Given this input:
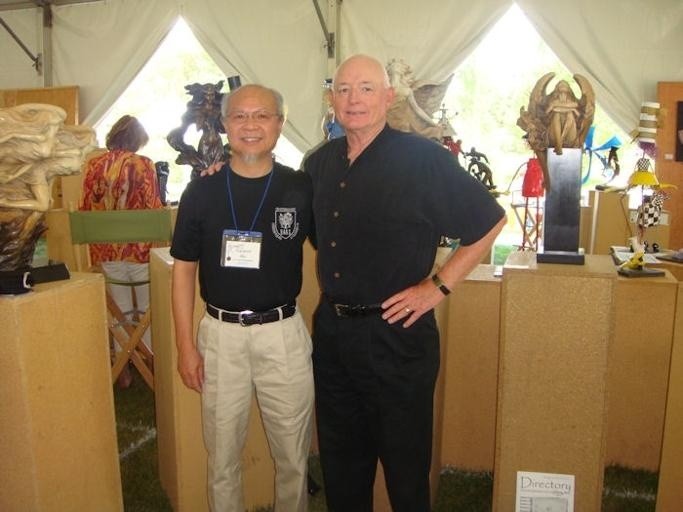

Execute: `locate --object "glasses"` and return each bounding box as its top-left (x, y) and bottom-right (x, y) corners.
top-left (227, 108), bottom-right (280, 122)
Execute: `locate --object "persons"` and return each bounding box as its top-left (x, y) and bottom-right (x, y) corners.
top-left (170, 83), bottom-right (317, 512)
top-left (515, 71), bottom-right (594, 193)
top-left (77, 115), bottom-right (164, 391)
top-left (170, 77), bottom-right (230, 182)
top-left (201, 54), bottom-right (507, 512)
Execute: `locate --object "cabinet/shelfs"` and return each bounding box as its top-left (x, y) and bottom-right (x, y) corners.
top-left (0, 272), bottom-right (127, 512)
top-left (148, 247), bottom-right (683, 511)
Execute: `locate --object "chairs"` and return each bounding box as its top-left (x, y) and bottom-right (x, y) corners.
top-left (70, 208), bottom-right (167, 394)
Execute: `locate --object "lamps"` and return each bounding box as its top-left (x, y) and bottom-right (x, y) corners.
top-left (618, 157), bottom-right (660, 245)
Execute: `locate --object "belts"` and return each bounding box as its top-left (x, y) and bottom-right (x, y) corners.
top-left (329, 301), bottom-right (384, 319)
top-left (205, 303), bottom-right (293, 327)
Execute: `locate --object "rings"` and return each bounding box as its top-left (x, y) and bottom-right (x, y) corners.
top-left (404, 307), bottom-right (411, 314)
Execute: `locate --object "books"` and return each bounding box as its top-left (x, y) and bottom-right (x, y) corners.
top-left (609, 245), bottom-right (666, 268)
top-left (656, 254), bottom-right (683, 263)
top-left (617, 267), bottom-right (666, 278)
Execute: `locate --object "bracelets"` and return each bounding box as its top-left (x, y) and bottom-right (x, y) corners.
top-left (432, 274), bottom-right (452, 295)
